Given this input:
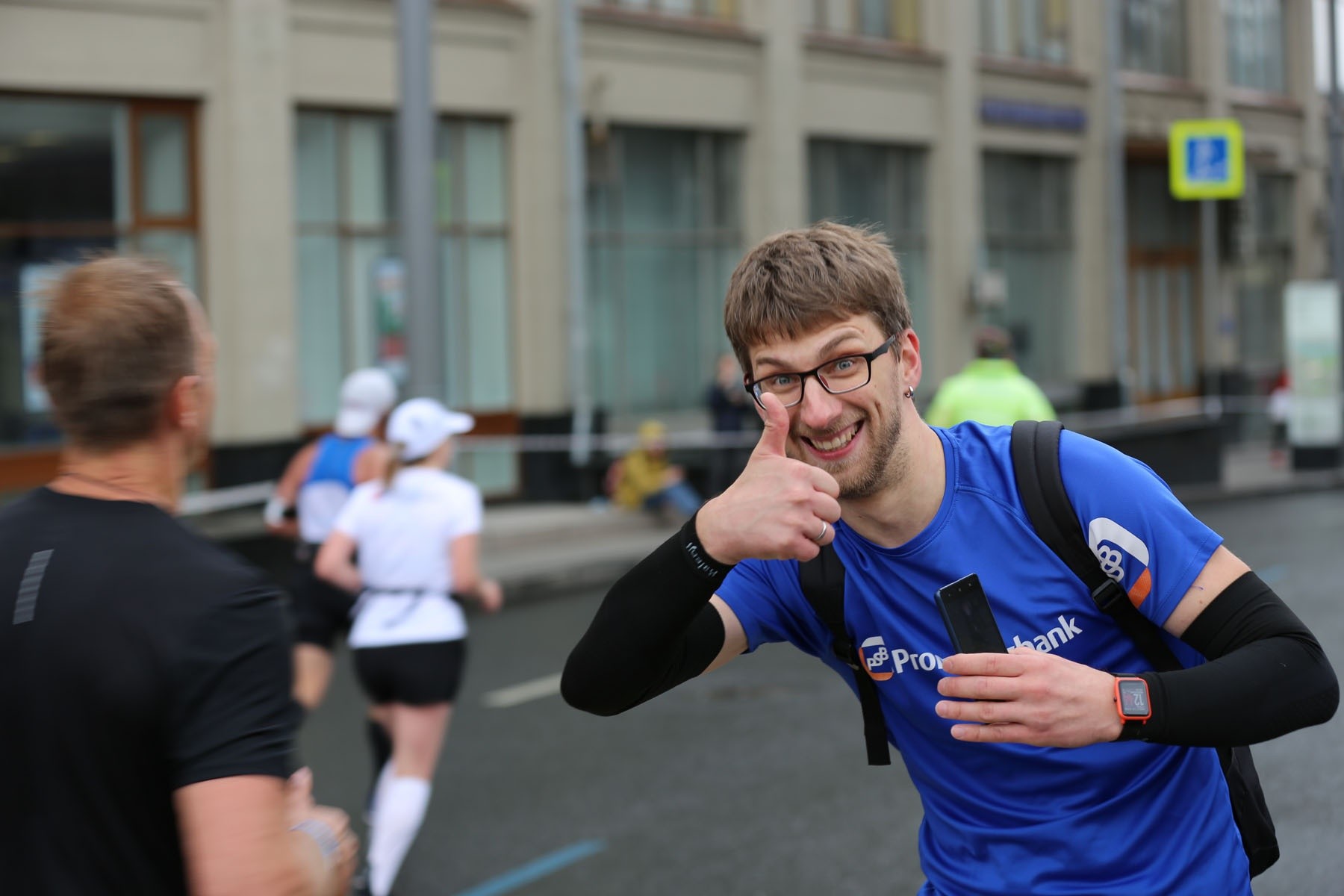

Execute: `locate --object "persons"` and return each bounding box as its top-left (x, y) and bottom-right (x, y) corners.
top-left (0, 257), bottom-right (363, 896)
top-left (707, 358), bottom-right (752, 482)
top-left (314, 397), bottom-right (502, 896)
top-left (922, 329), bottom-right (1061, 428)
top-left (559, 220), bottom-right (1340, 896)
top-left (262, 366), bottom-right (395, 822)
top-left (614, 420), bottom-right (701, 518)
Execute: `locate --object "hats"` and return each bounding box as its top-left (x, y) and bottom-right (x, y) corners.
top-left (332, 367), bottom-right (397, 438)
top-left (385, 398), bottom-right (476, 465)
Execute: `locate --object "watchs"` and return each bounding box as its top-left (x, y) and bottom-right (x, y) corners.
top-left (1114, 670), bottom-right (1153, 743)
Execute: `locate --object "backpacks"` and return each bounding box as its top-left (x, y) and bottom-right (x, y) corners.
top-left (799, 419), bottom-right (1284, 881)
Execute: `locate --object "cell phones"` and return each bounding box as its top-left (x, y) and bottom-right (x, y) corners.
top-left (933, 573), bottom-right (1010, 703)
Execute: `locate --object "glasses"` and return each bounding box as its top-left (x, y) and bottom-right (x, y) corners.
top-left (743, 334), bottom-right (896, 411)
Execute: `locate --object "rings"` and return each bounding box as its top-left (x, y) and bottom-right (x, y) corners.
top-left (814, 522), bottom-right (829, 542)
